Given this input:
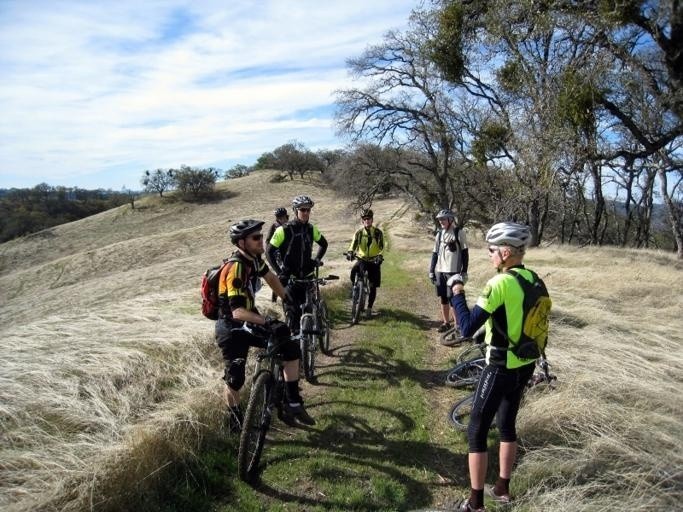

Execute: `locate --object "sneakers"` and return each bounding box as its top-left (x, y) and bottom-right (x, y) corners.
top-left (283, 403), bottom-right (315, 425)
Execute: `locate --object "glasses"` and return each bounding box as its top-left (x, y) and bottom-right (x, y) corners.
top-left (300, 208), bottom-right (310, 212)
top-left (253, 235), bottom-right (263, 240)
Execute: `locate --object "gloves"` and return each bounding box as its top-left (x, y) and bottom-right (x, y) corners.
top-left (447, 274), bottom-right (463, 288)
top-left (266, 320), bottom-right (291, 337)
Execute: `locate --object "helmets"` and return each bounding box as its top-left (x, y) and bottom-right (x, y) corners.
top-left (436, 209), bottom-right (453, 223)
top-left (361, 209), bottom-right (373, 216)
top-left (486, 222), bottom-right (530, 248)
top-left (293, 196), bottom-right (313, 212)
top-left (230, 220), bottom-right (265, 237)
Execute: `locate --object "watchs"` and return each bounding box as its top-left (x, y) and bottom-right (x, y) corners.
top-left (263, 315), bottom-right (271, 329)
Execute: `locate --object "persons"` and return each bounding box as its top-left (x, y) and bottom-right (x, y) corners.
top-left (428, 209), bottom-right (471, 332)
top-left (216, 219), bottom-right (320, 433)
top-left (263, 194), bottom-right (329, 371)
top-left (446, 220), bottom-right (540, 511)
top-left (265, 206), bottom-right (294, 304)
top-left (347, 207), bottom-right (384, 320)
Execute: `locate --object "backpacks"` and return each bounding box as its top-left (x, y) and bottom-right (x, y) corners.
top-left (494, 268), bottom-right (551, 359)
top-left (201, 257), bottom-right (242, 320)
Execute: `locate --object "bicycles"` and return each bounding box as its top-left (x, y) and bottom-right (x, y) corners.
top-left (440, 326), bottom-right (487, 366)
top-left (445, 351), bottom-right (558, 429)
top-left (343, 252), bottom-right (384, 323)
top-left (287, 275), bottom-right (339, 382)
top-left (270, 252), bottom-right (289, 318)
top-left (231, 323), bottom-right (326, 481)
